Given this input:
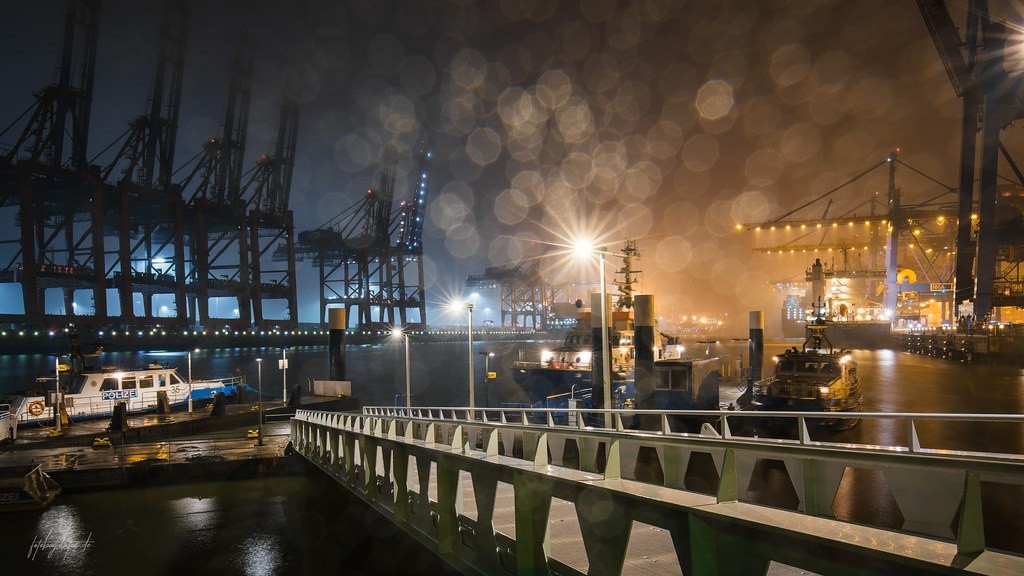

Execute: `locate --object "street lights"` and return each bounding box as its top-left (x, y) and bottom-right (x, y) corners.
top-left (444, 296), bottom-right (476, 421)
top-left (572, 242), bottom-right (613, 429)
top-left (480, 351), bottom-right (497, 407)
top-left (386, 325), bottom-right (412, 418)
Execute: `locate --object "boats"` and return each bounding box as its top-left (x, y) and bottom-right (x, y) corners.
top-left (750, 296), bottom-right (864, 435)
top-left (0, 306), bottom-right (356, 448)
top-left (511, 238), bottom-right (685, 430)
top-left (538, 292), bottom-right (767, 465)
top-left (0, 354), bottom-right (250, 428)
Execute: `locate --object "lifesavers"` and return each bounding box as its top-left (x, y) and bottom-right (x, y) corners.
top-left (28, 401), bottom-right (45, 415)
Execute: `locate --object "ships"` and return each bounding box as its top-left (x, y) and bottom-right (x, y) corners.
top-left (730, 2), bottom-right (1023, 367)
